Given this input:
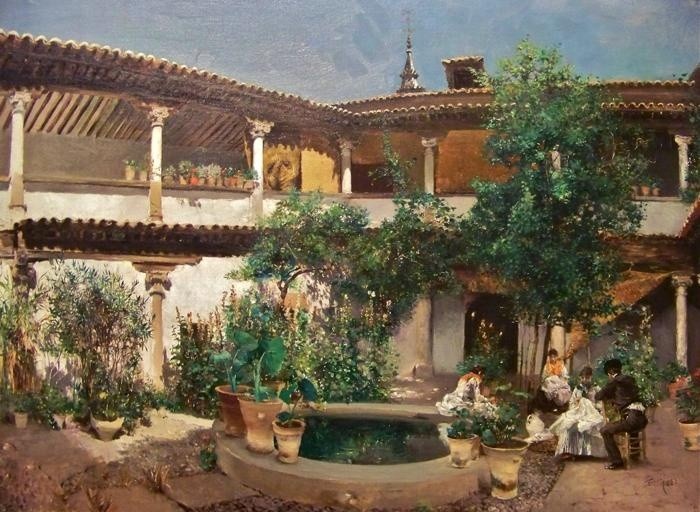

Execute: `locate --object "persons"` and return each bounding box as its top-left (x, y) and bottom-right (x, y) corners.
top-left (542, 349), bottom-right (569, 412)
top-left (594, 358), bottom-right (648, 470)
top-left (436, 366), bottom-right (500, 419)
top-left (550, 366), bottom-right (610, 460)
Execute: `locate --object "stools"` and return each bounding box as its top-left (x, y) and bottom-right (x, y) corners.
top-left (617, 429), bottom-right (646, 469)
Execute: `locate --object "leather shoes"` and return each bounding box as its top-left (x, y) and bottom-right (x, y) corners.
top-left (604, 463), bottom-right (624, 470)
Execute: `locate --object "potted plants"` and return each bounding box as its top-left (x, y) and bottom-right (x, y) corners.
top-left (455, 349), bottom-right (514, 397)
top-left (0, 259), bottom-right (154, 442)
top-left (473, 404), bottom-right (529, 500)
top-left (215, 322), bottom-right (318, 466)
top-left (676, 385), bottom-right (700, 451)
top-left (123, 159), bottom-right (256, 193)
top-left (663, 360), bottom-right (688, 400)
top-left (626, 170), bottom-right (661, 196)
top-left (444, 407), bottom-right (477, 468)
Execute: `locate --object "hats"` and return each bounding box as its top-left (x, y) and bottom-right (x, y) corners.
top-left (579, 367), bottom-right (593, 377)
top-left (604, 359), bottom-right (621, 375)
